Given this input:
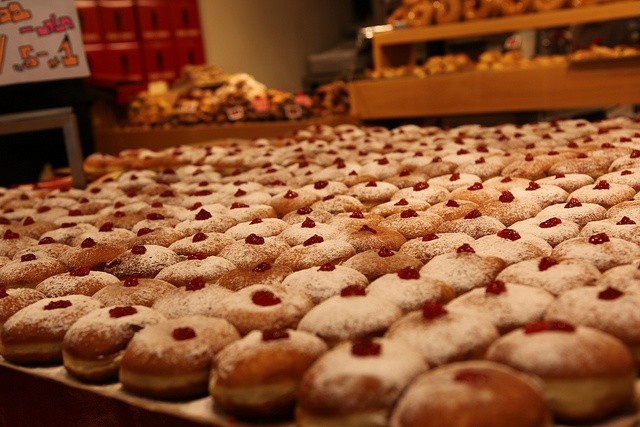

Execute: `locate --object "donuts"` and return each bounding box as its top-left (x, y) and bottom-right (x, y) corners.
top-left (387, 0), bottom-right (608, 29)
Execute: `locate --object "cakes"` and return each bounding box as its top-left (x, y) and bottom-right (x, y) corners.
top-left (0, 118), bottom-right (639, 427)
top-left (129, 59), bottom-right (347, 124)
top-left (361, 41), bottom-right (639, 81)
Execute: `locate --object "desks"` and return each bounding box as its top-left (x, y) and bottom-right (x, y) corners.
top-left (2, 104), bottom-right (84, 189)
